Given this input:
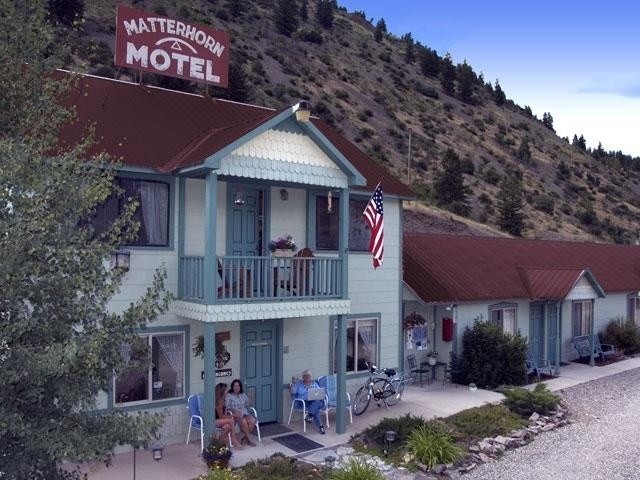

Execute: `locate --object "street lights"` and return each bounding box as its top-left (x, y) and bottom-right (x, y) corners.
top-left (133, 431), bottom-right (164, 480)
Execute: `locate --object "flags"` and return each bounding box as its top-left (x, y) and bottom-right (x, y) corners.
top-left (363, 182), bottom-right (388, 270)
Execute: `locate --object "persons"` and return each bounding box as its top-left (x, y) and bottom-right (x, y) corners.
top-left (226, 379), bottom-right (260, 446)
top-left (289, 369), bottom-right (327, 436)
top-left (212, 382), bottom-right (246, 450)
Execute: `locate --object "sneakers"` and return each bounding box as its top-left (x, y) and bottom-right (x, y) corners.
top-left (320, 425), bottom-right (326, 434)
top-left (305, 414), bottom-right (313, 423)
top-left (234, 437), bottom-right (256, 450)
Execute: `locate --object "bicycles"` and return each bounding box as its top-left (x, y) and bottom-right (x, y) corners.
top-left (351, 358), bottom-right (407, 417)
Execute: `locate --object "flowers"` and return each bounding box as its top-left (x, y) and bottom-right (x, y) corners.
top-left (269, 235), bottom-right (297, 251)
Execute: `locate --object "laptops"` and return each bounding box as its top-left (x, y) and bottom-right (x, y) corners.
top-left (304, 388), bottom-right (325, 401)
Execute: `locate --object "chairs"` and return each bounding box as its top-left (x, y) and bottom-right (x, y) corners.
top-left (523, 350), bottom-right (553, 379)
top-left (573, 334), bottom-right (616, 364)
top-left (407, 354), bottom-right (464, 390)
top-left (185, 392), bottom-right (261, 453)
top-left (216, 247), bottom-right (317, 300)
top-left (287, 374), bottom-right (353, 433)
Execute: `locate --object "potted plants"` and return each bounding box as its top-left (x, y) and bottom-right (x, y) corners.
top-left (425, 350), bottom-right (439, 366)
